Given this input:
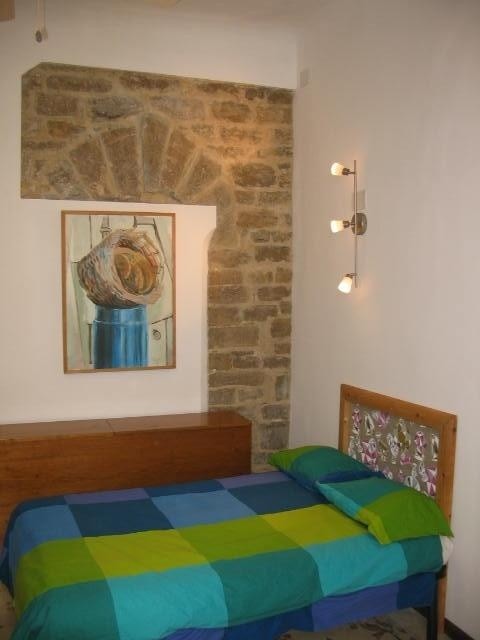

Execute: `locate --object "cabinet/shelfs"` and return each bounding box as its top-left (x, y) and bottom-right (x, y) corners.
top-left (0, 410), bottom-right (253, 538)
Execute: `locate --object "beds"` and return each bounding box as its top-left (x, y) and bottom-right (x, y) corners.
top-left (0, 384), bottom-right (458, 638)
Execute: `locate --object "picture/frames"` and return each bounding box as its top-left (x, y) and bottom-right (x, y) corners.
top-left (61, 210), bottom-right (176, 373)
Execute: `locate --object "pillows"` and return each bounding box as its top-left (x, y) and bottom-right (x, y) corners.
top-left (264, 445), bottom-right (367, 490)
top-left (316, 471), bottom-right (455, 545)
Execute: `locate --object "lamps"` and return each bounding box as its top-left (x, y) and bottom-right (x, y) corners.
top-left (330, 160), bottom-right (358, 294)
